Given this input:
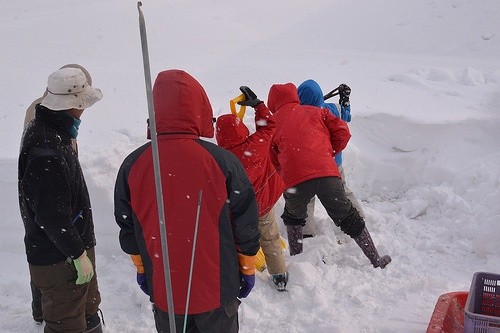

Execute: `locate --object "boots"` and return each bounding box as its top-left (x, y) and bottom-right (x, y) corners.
top-left (353, 225), bottom-right (392, 270)
top-left (286, 224), bottom-right (304, 256)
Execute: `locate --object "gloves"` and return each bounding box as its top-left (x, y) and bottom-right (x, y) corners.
top-left (236, 251), bottom-right (257, 299)
top-left (130, 254), bottom-right (150, 295)
top-left (238, 85), bottom-right (261, 108)
top-left (73, 249), bottom-right (95, 286)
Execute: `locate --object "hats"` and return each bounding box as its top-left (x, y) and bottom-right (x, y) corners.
top-left (40, 67), bottom-right (104, 111)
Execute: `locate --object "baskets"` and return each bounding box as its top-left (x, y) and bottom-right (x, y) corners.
top-left (464, 271), bottom-right (500, 333)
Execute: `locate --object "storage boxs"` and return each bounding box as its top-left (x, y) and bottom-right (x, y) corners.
top-left (463, 271), bottom-right (500, 333)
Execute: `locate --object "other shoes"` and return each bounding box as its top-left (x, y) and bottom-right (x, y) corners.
top-left (273, 271), bottom-right (288, 292)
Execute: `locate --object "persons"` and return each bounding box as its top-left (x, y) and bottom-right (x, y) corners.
top-left (18, 63), bottom-right (103, 333)
top-left (268, 82), bottom-right (392, 268)
top-left (114, 68), bottom-right (260, 333)
top-left (298, 80), bottom-right (366, 238)
top-left (214, 86), bottom-right (289, 292)
